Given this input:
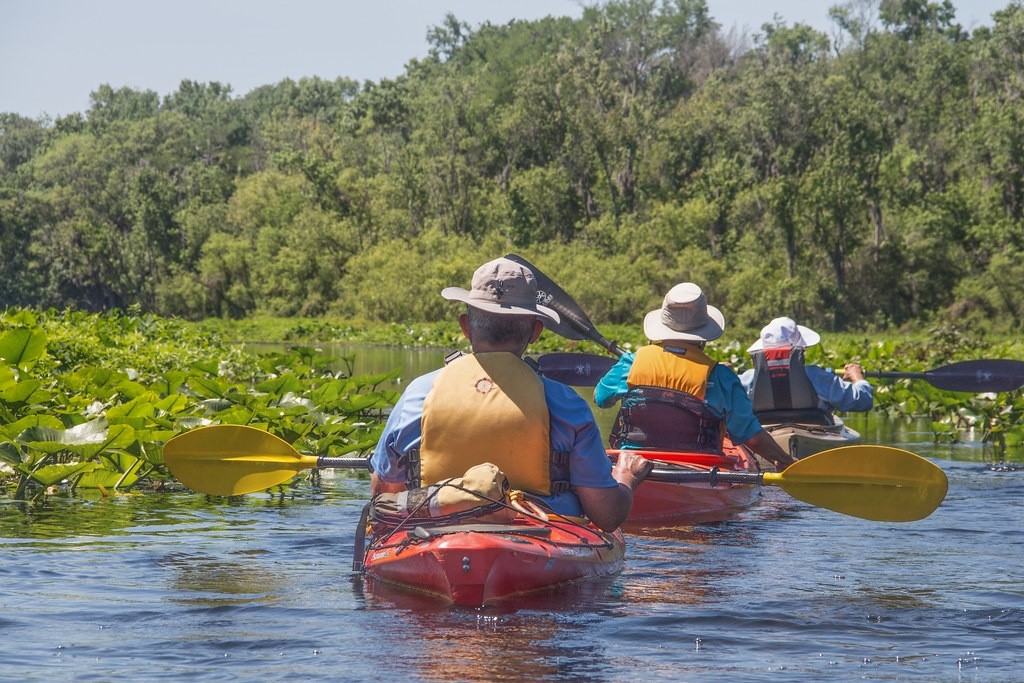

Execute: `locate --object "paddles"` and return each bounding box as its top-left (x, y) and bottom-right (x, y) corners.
top-left (502, 253), bottom-right (627, 361)
top-left (160, 422), bottom-right (952, 524)
top-left (539, 350), bottom-right (1024, 398)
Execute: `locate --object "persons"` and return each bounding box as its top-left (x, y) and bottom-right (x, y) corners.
top-left (369, 257), bottom-right (653, 533)
top-left (737, 316), bottom-right (874, 413)
top-left (593, 282), bottom-right (799, 474)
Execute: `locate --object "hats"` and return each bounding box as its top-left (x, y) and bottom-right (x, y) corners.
top-left (644, 282), bottom-right (725, 340)
top-left (746, 315), bottom-right (820, 353)
top-left (441, 258), bottom-right (561, 325)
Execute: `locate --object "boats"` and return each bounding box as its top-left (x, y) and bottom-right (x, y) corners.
top-left (602, 435), bottom-right (765, 528)
top-left (753, 412), bottom-right (863, 471)
top-left (352, 464), bottom-right (628, 611)
